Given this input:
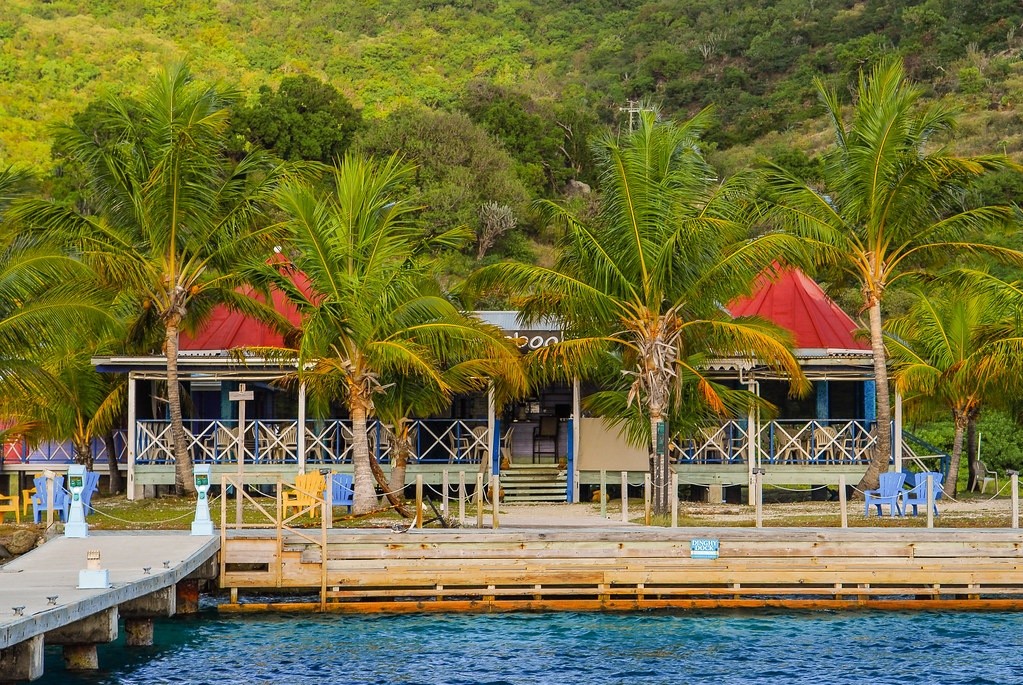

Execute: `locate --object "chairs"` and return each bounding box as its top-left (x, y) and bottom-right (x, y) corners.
top-left (446, 426), bottom-right (471, 464)
top-left (970, 460), bottom-right (998, 495)
top-left (22, 474), bottom-right (62, 516)
top-left (152, 426), bottom-right (391, 467)
top-left (282, 471), bottom-right (353, 518)
top-left (677, 423), bottom-right (878, 464)
top-left (531, 415), bottom-right (559, 463)
top-left (58, 472), bottom-right (100, 522)
top-left (500, 425), bottom-right (515, 464)
top-left (863, 472), bottom-right (908, 518)
top-left (473, 426), bottom-right (488, 464)
top-left (31, 476), bottom-right (69, 524)
top-left (900, 471), bottom-right (943, 518)
top-left (0, 494), bottom-right (20, 524)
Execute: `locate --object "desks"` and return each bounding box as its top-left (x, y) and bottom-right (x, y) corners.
top-left (460, 433), bottom-right (472, 437)
top-left (193, 434), bottom-right (212, 464)
